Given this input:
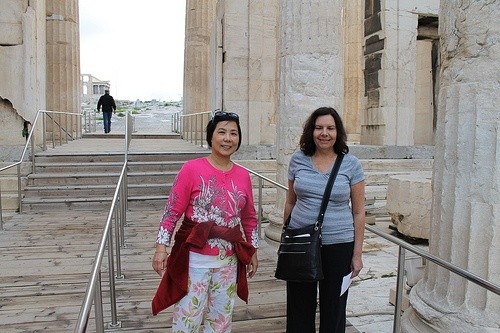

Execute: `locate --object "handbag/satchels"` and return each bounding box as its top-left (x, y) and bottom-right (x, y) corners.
top-left (275, 224), bottom-right (324, 281)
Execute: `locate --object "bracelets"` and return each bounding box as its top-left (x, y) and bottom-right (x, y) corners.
top-left (154, 251), bottom-right (167, 254)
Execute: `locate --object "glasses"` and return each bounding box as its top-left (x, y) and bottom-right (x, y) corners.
top-left (212, 110), bottom-right (239, 122)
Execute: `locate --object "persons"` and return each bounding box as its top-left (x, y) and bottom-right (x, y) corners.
top-left (152, 110), bottom-right (260, 333)
top-left (282, 107), bottom-right (365, 333)
top-left (96, 90), bottom-right (116, 133)
